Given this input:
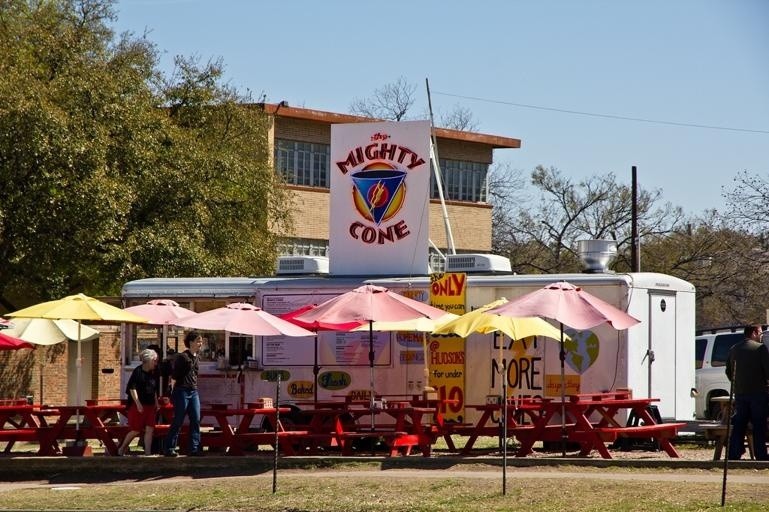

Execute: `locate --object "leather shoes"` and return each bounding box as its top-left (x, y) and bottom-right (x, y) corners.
top-left (728, 457), bottom-right (740, 459)
top-left (756, 454), bottom-right (769, 460)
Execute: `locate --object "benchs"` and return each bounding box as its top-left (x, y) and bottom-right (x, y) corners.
top-left (0, 422), bottom-right (689, 460)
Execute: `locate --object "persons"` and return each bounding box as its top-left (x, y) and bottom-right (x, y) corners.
top-left (725, 322), bottom-right (769, 461)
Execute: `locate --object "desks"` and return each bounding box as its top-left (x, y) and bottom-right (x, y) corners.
top-left (0, 391), bottom-right (681, 458)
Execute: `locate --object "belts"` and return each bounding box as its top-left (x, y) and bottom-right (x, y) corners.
top-left (176, 384), bottom-right (198, 387)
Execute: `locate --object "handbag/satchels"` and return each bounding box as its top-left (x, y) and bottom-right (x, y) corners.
top-left (169, 390), bottom-right (177, 404)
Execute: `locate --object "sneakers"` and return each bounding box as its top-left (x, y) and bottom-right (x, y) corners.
top-left (164, 450), bottom-right (179, 456)
top-left (189, 450), bottom-right (205, 456)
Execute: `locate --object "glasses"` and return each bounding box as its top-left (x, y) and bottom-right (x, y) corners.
top-left (757, 330), bottom-right (763, 336)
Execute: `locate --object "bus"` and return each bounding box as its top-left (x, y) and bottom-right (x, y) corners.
top-left (121, 239), bottom-right (696, 448)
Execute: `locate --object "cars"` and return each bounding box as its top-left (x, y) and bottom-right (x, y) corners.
top-left (696, 324), bottom-right (769, 421)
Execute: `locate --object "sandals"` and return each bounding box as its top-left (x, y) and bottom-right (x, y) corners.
top-left (145, 454), bottom-right (155, 456)
top-left (119, 449), bottom-right (126, 455)
